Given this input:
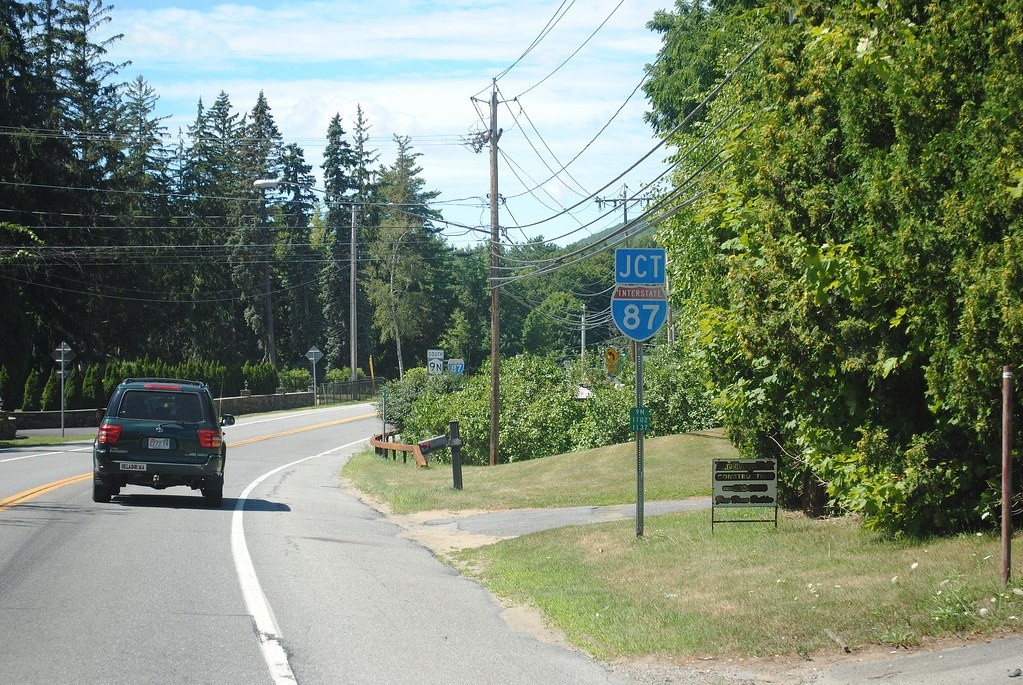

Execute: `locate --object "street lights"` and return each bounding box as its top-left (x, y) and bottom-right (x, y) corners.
top-left (254, 178), bottom-right (358, 393)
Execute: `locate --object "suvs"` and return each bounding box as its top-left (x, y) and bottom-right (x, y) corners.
top-left (93, 376), bottom-right (235, 506)
